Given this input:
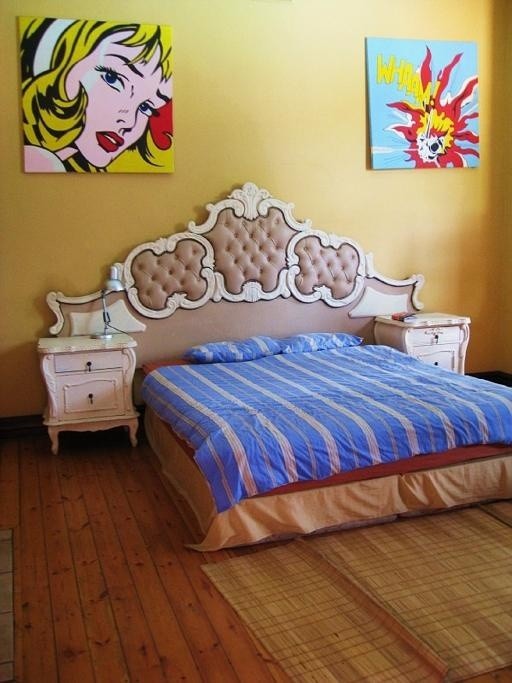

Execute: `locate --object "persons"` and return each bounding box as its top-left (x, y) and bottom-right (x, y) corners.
top-left (18, 16), bottom-right (175, 175)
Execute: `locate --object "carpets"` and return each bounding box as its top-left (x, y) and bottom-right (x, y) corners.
top-left (198, 502), bottom-right (512, 683)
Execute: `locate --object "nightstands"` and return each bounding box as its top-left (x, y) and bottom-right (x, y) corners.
top-left (35, 333), bottom-right (141, 454)
top-left (374, 312), bottom-right (471, 375)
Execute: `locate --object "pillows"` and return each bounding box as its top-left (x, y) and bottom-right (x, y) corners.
top-left (182, 335), bottom-right (288, 364)
top-left (272, 332), bottom-right (368, 354)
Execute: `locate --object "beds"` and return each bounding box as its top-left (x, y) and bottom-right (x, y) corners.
top-left (45, 181), bottom-right (512, 553)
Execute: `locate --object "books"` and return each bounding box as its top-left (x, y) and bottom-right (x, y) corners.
top-left (390, 310), bottom-right (423, 323)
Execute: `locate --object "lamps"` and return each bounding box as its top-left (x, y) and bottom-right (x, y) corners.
top-left (90, 279), bottom-right (126, 340)
